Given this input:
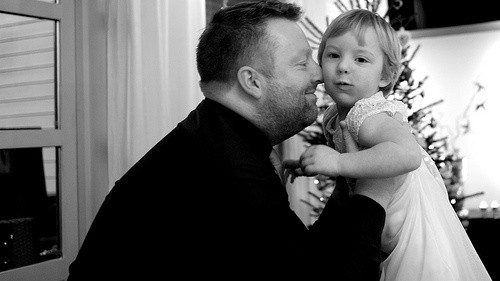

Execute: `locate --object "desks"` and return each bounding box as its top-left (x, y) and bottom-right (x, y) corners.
top-left (456, 209), bottom-right (500, 281)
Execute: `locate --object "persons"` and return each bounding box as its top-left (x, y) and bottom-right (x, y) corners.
top-left (280, 10), bottom-right (493, 281)
top-left (67, 0), bottom-right (411, 281)
